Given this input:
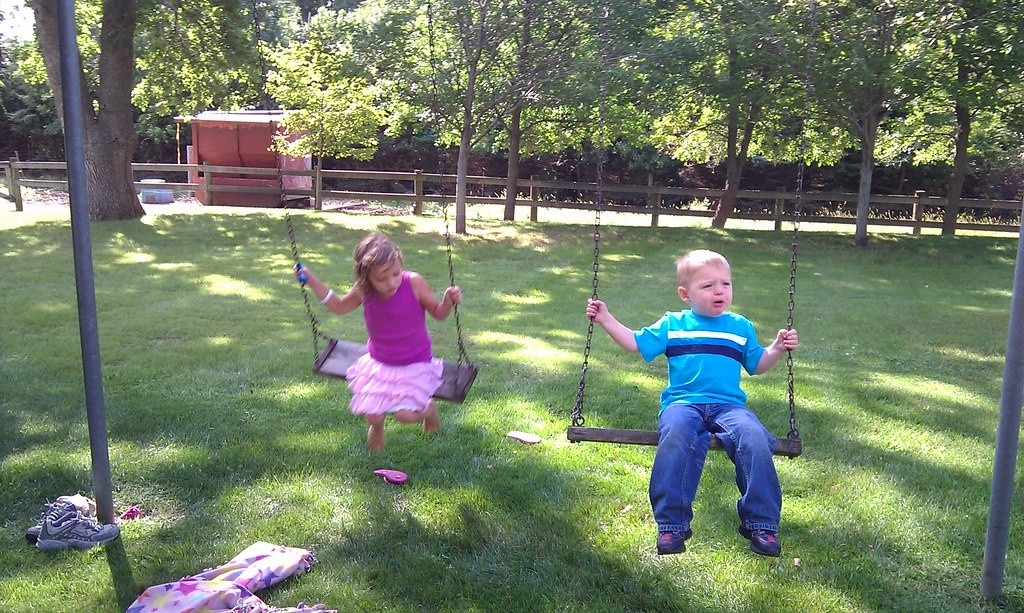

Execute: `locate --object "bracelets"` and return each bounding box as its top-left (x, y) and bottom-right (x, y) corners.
top-left (320, 289), bottom-right (333, 305)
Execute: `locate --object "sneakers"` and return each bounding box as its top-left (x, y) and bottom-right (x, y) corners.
top-left (738, 525), bottom-right (781, 557)
top-left (657, 528), bottom-right (692, 554)
top-left (26, 497), bottom-right (95, 540)
top-left (35, 501), bottom-right (120, 551)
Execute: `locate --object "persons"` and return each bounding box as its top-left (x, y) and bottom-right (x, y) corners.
top-left (293, 231), bottom-right (462, 450)
top-left (586, 249), bottom-right (798, 557)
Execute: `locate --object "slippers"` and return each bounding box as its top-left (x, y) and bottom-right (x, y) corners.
top-left (374, 469), bottom-right (408, 485)
top-left (507, 431), bottom-right (542, 445)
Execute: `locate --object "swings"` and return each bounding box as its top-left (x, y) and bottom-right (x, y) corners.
top-left (252, 1), bottom-right (478, 403)
top-left (565, 0), bottom-right (806, 458)
